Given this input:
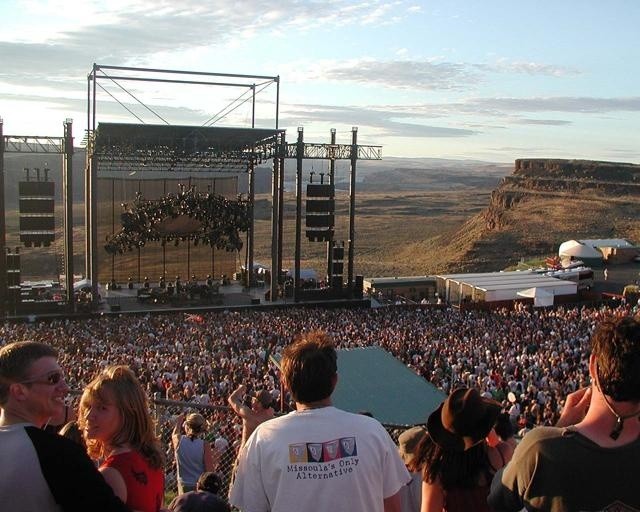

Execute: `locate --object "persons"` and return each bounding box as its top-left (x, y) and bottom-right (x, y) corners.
top-left (603, 267), bottom-right (608, 280)
top-left (1, 276), bottom-right (640, 510)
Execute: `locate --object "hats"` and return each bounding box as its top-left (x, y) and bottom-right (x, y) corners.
top-left (426, 387), bottom-right (504, 450)
top-left (397, 426), bottom-right (432, 466)
top-left (197, 472), bottom-right (222, 493)
top-left (249, 389), bottom-right (273, 406)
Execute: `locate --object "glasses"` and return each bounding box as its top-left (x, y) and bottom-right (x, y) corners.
top-left (21, 369), bottom-right (62, 385)
